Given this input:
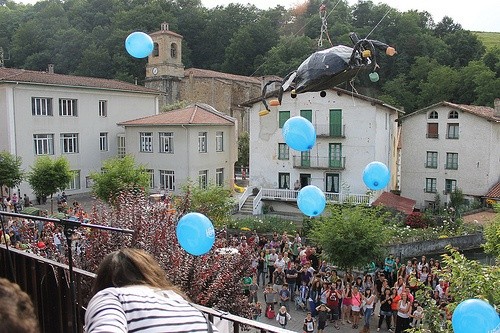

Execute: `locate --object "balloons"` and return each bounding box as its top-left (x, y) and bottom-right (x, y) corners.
top-left (453, 293), bottom-right (496, 333)
top-left (173, 213), bottom-right (216, 257)
top-left (282, 116), bottom-right (318, 151)
top-left (296, 185), bottom-right (326, 218)
top-left (126, 32), bottom-right (155, 59)
top-left (363, 161), bottom-right (390, 194)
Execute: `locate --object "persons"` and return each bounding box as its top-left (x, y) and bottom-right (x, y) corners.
top-left (363, 288), bottom-right (375, 333)
top-left (277, 305), bottom-right (294, 330)
top-left (242, 170), bottom-right (246, 181)
top-left (0, 187), bottom-right (94, 267)
top-left (84, 248), bottom-right (219, 332)
top-left (293, 179), bottom-right (301, 201)
top-left (0, 279), bottom-right (41, 333)
top-left (215, 223), bottom-right (453, 333)
top-left (350, 285), bottom-right (362, 328)
top-left (378, 289), bottom-right (392, 333)
top-left (309, 271), bottom-right (349, 333)
top-left (162, 195), bottom-right (172, 214)
top-left (301, 312), bottom-right (317, 333)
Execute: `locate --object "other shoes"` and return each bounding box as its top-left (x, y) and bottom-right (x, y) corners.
top-left (325, 320), bottom-right (397, 333)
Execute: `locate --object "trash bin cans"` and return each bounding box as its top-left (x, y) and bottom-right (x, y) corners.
top-left (47, 213), bottom-right (65, 225)
top-left (68, 215), bottom-right (89, 227)
top-left (20, 207), bottom-right (41, 216)
